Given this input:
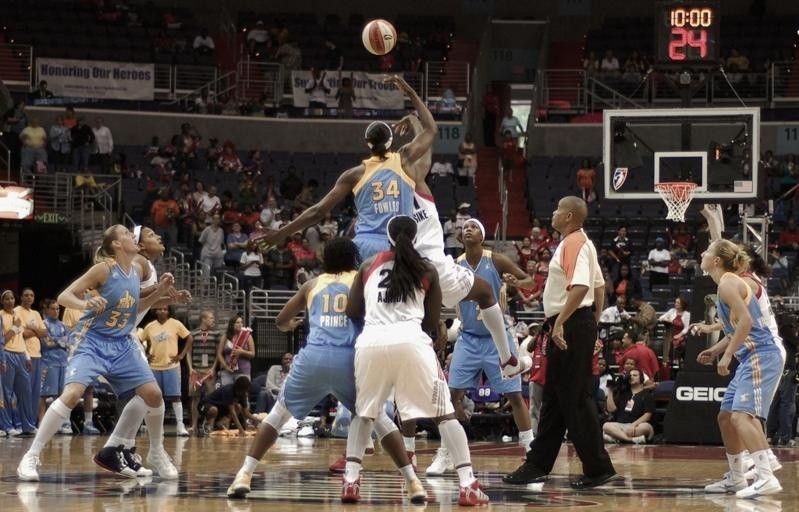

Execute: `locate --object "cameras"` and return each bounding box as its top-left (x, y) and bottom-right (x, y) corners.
top-left (605, 368), bottom-right (630, 389)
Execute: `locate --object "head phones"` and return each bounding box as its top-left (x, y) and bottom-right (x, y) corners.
top-left (641, 376), bottom-right (644, 382)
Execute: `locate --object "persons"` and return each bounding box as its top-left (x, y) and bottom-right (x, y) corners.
top-left (218, 315), bottom-right (255, 408)
top-left (335, 68), bottom-right (356, 118)
top-left (689, 243), bottom-right (787, 480)
top-left (197, 377), bottom-right (262, 437)
top-left (499, 130), bottom-right (516, 182)
top-left (457, 132), bottom-right (478, 188)
top-left (599, 295), bottom-right (629, 324)
top-left (779, 219), bottom-right (799, 252)
top-left (190, 20), bottom-right (397, 74)
top-left (636, 337), bottom-right (660, 380)
top-left (624, 357), bottom-right (656, 390)
top-left (499, 108), bottom-right (524, 145)
top-left (448, 217), bottom-right (535, 456)
top-left (390, 112), bottom-right (533, 379)
top-left (13, 288), bottom-right (48, 425)
top-left (501, 196), bottom-right (616, 490)
top-left (618, 331), bottom-right (638, 371)
top-left (139, 122), bottom-right (338, 290)
top-left (1, 99), bottom-right (113, 173)
top-left (659, 297), bottom-right (690, 357)
top-left (513, 218), bottom-right (560, 313)
top-left (92, 224), bottom-right (192, 479)
top-left (226, 238), bottom-right (427, 501)
top-left (186, 310), bottom-right (219, 432)
top-left (255, 352), bottom-right (293, 416)
top-left (603, 368), bottom-right (656, 444)
top-left (37, 299), bottom-right (68, 428)
top-left (575, 158), bottom-right (596, 201)
top-left (526, 323), bottom-right (547, 419)
top-left (32, 80), bottom-right (53, 105)
top-left (62, 292), bottom-right (100, 435)
top-left (480, 83), bottom-right (498, 146)
top-left (0, 289), bottom-right (39, 436)
top-left (140, 304), bottom-right (192, 437)
top-left (194, 87), bottom-right (209, 112)
top-left (648, 236), bottom-right (671, 291)
top-left (608, 226), bottom-right (634, 265)
top-left (254, 75), bottom-right (437, 267)
top-left (0, 315), bottom-right (7, 436)
top-left (623, 294), bottom-right (657, 340)
top-left (18, 225), bottom-right (179, 481)
top-left (583, 46), bottom-right (750, 98)
top-left (696, 239), bottom-right (783, 499)
top-left (613, 263), bottom-right (643, 302)
top-left (329, 418), bottom-right (418, 474)
top-left (304, 67), bottom-right (331, 108)
top-left (341, 214), bottom-right (490, 507)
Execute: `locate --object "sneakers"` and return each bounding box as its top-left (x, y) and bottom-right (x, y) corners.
top-left (632, 435), bottom-right (646, 444)
top-left (227, 476), bottom-right (252, 497)
top-left (426, 448), bottom-right (454, 476)
top-left (341, 474), bottom-right (361, 502)
top-left (747, 459), bottom-right (782, 479)
top-left (406, 480), bottom-right (427, 500)
top-left (406, 451), bottom-right (417, 472)
top-left (17, 451), bottom-right (39, 481)
top-left (59, 422), bottom-right (74, 434)
top-left (93, 446), bottom-right (137, 479)
top-left (146, 446), bottom-right (179, 480)
top-left (458, 478), bottom-right (490, 505)
top-left (722, 458), bottom-right (755, 478)
top-left (83, 421), bottom-right (100, 435)
top-left (177, 423), bottom-right (189, 436)
top-left (123, 447), bottom-right (152, 476)
top-left (704, 470), bottom-right (749, 494)
top-left (329, 453), bottom-right (363, 472)
top-left (735, 468), bottom-right (783, 498)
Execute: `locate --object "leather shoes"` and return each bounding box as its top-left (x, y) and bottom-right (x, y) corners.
top-left (502, 461), bottom-right (551, 484)
top-left (571, 470), bottom-right (616, 488)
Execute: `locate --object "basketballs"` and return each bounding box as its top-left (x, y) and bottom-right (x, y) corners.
top-left (362, 19), bottom-right (397, 56)
